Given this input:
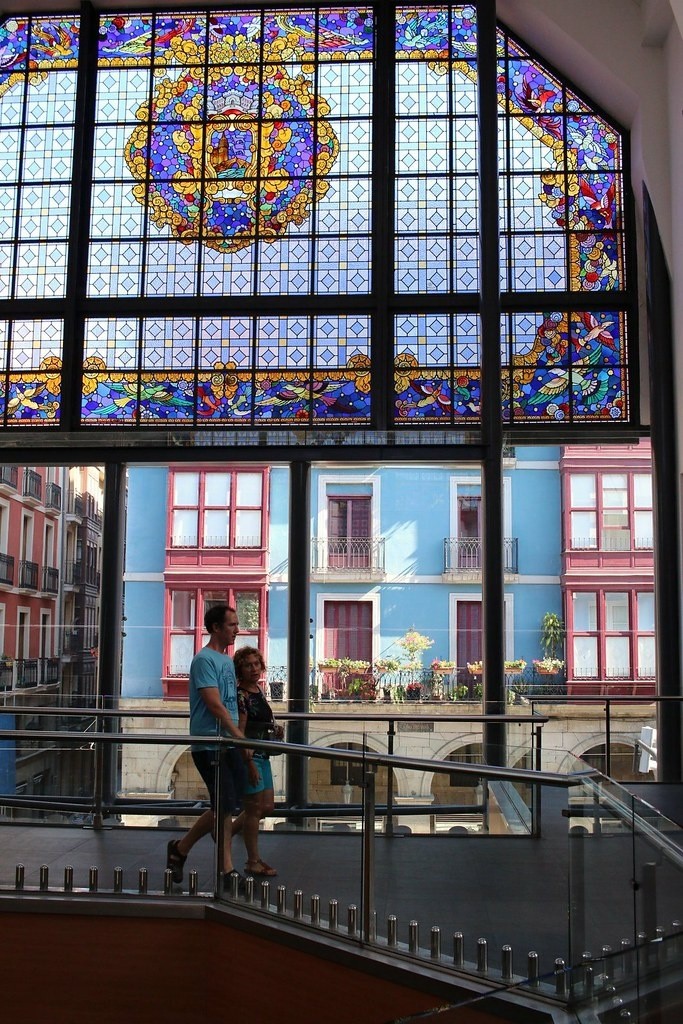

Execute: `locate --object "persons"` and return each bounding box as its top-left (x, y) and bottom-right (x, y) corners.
top-left (210, 646), bottom-right (281, 878)
top-left (165, 605), bottom-right (264, 899)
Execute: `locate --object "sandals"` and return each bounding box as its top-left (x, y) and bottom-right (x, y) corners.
top-left (244, 859), bottom-right (277, 876)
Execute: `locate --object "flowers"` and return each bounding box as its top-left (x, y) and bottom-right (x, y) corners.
top-left (315, 628), bottom-right (563, 702)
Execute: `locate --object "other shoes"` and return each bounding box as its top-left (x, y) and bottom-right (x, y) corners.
top-left (225, 869), bottom-right (257, 892)
top-left (166, 839), bottom-right (187, 883)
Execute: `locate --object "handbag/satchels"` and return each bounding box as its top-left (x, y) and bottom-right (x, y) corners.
top-left (266, 723), bottom-right (285, 756)
